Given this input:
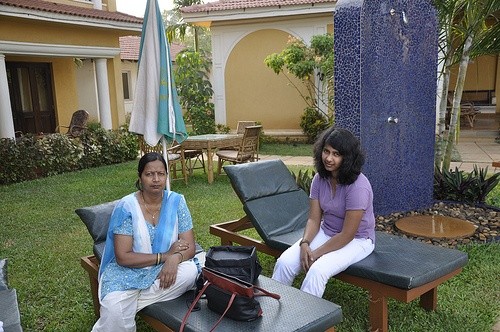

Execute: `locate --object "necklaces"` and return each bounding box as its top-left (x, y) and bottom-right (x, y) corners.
top-left (141, 191), bottom-right (161, 218)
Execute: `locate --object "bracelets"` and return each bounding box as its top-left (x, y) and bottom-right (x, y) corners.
top-left (156, 253), bottom-right (161, 266)
top-left (173, 251), bottom-right (184, 263)
top-left (299, 238), bottom-right (310, 245)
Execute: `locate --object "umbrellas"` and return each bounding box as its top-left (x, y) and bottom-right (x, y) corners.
top-left (128, 0), bottom-right (188, 191)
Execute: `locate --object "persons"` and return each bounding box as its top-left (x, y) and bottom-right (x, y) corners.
top-left (272, 127), bottom-right (375, 298)
top-left (91, 152), bottom-right (198, 332)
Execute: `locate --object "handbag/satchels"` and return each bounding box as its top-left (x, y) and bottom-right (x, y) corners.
top-left (178, 267), bottom-right (280, 332)
top-left (206, 245), bottom-right (262, 284)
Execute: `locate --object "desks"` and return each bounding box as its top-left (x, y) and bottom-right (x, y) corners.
top-left (173, 134), bottom-right (245, 184)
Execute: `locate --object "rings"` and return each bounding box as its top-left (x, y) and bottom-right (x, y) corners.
top-left (178, 240), bottom-right (182, 251)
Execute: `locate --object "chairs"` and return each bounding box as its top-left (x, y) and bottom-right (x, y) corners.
top-left (75, 199), bottom-right (342, 332)
top-left (173, 150), bottom-right (206, 177)
top-left (219, 121), bottom-right (257, 150)
top-left (141, 140), bottom-right (188, 186)
top-left (447, 96), bottom-right (479, 128)
top-left (209, 159), bottom-right (468, 332)
top-left (54, 110), bottom-right (89, 139)
top-left (216, 125), bottom-right (262, 175)
